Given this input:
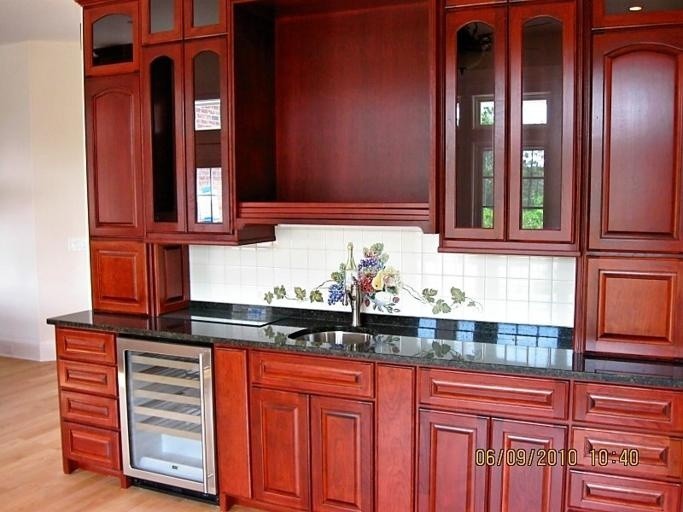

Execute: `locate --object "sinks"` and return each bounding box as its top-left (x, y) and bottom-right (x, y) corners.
top-left (286, 324), bottom-right (379, 346)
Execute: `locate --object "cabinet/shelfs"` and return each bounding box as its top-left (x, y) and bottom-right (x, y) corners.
top-left (140, 1), bottom-right (276, 247)
top-left (233, 0), bottom-right (438, 234)
top-left (214, 345), bottom-right (416, 512)
top-left (566, 381), bottom-right (682, 511)
top-left (438, 2), bottom-right (586, 257)
top-left (573, 2), bottom-right (681, 359)
top-left (55, 326), bottom-right (128, 490)
top-left (74, 0), bottom-right (190, 316)
top-left (415, 367), bottom-right (570, 511)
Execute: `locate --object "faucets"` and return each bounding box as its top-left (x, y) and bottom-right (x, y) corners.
top-left (350, 283), bottom-right (363, 328)
